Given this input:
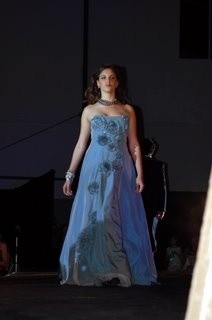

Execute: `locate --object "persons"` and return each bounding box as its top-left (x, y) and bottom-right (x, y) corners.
top-left (0, 241), bottom-right (9, 276)
top-left (133, 138), bottom-right (167, 253)
top-left (167, 236), bottom-right (185, 272)
top-left (56, 63), bottom-right (159, 287)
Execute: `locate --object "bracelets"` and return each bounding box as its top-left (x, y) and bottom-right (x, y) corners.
top-left (65, 171), bottom-right (75, 182)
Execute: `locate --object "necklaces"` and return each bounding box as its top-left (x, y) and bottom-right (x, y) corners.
top-left (99, 99), bottom-right (119, 106)
